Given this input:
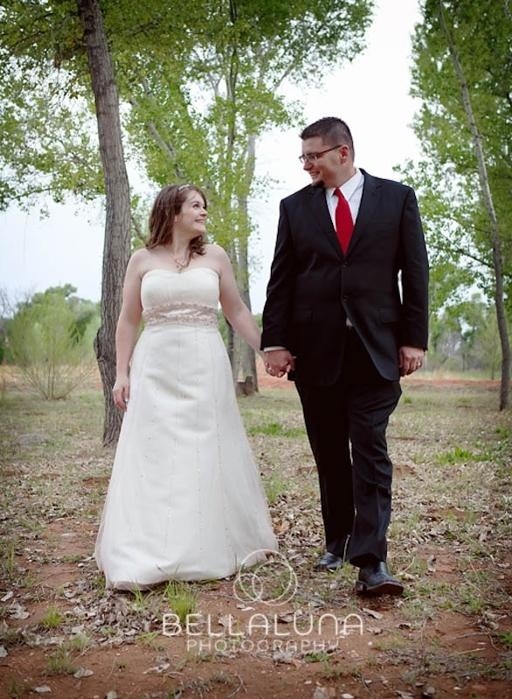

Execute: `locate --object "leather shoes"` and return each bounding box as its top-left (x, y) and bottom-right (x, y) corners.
top-left (356, 561), bottom-right (403, 595)
top-left (313, 552), bottom-right (344, 573)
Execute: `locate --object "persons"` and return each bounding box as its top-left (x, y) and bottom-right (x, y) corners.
top-left (94, 183), bottom-right (298, 591)
top-left (261, 117), bottom-right (429, 597)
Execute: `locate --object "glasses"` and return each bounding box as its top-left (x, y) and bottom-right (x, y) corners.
top-left (299, 145), bottom-right (342, 163)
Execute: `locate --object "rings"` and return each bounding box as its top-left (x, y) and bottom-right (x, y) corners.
top-left (417, 363), bottom-right (421, 366)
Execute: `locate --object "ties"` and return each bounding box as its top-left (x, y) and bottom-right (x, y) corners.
top-left (334, 187), bottom-right (356, 259)
